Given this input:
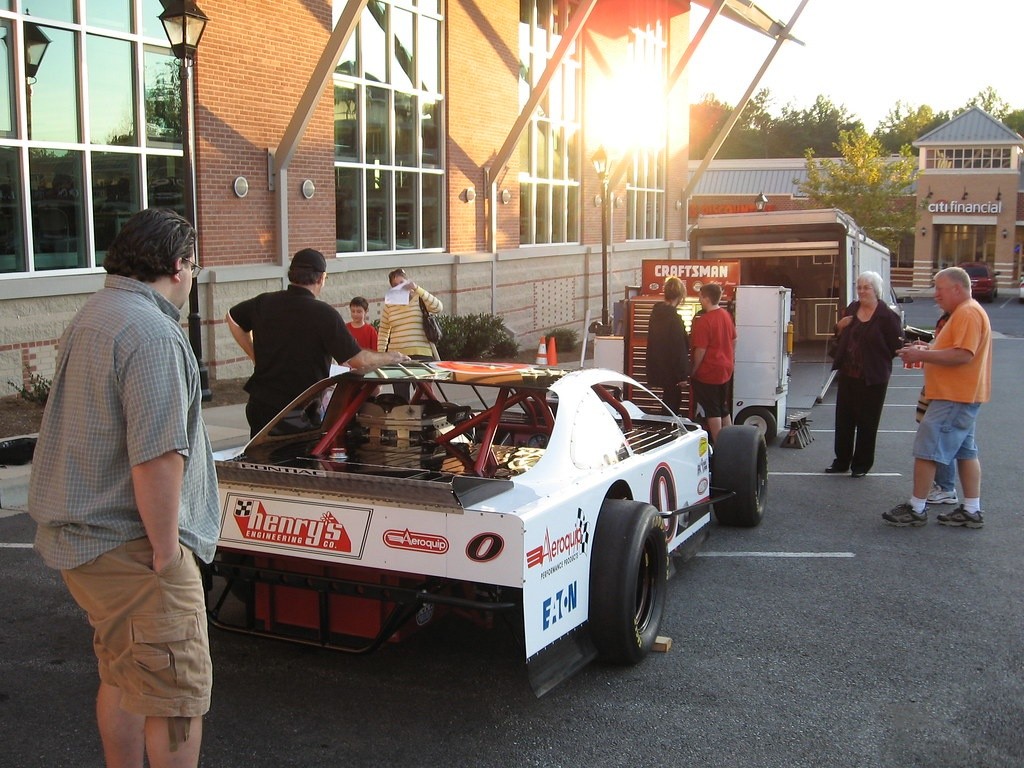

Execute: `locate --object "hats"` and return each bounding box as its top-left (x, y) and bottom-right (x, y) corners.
top-left (292, 248), bottom-right (326, 272)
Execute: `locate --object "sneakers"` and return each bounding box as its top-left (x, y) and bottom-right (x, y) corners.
top-left (882, 503), bottom-right (928, 527)
top-left (926, 481), bottom-right (958, 503)
top-left (937, 504), bottom-right (984, 529)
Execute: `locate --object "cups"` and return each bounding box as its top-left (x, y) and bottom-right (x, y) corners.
top-left (912, 345), bottom-right (927, 369)
top-left (903, 342), bottom-right (916, 369)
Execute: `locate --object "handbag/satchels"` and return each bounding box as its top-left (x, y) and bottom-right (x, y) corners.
top-left (419, 296), bottom-right (442, 343)
top-left (828, 338), bottom-right (838, 358)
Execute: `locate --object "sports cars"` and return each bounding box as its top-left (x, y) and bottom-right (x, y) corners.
top-left (186, 359), bottom-right (770, 701)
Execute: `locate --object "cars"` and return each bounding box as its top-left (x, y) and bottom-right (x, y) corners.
top-left (958, 262), bottom-right (1002, 304)
top-left (1018, 273), bottom-right (1024, 304)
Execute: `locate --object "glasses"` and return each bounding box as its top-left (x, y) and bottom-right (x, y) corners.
top-left (182, 257), bottom-right (202, 278)
top-left (389, 268), bottom-right (405, 278)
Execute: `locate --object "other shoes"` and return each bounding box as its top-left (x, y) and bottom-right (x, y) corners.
top-left (852, 472), bottom-right (865, 477)
top-left (825, 467), bottom-right (849, 472)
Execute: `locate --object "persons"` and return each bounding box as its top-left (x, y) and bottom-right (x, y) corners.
top-left (689, 284), bottom-right (737, 446)
top-left (881, 267), bottom-right (991, 529)
top-left (321, 296), bottom-right (382, 422)
top-left (825, 271), bottom-right (902, 479)
top-left (226, 248), bottom-right (411, 443)
top-left (377, 268), bottom-right (443, 402)
top-left (28, 207), bottom-right (220, 768)
top-left (645, 278), bottom-right (692, 416)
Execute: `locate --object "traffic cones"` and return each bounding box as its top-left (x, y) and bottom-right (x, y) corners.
top-left (534, 336), bottom-right (548, 366)
top-left (547, 336), bottom-right (557, 367)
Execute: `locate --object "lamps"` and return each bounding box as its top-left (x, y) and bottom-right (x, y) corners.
top-left (302, 179), bottom-right (316, 199)
top-left (234, 176), bottom-right (249, 198)
top-left (465, 187), bottom-right (477, 202)
top-left (614, 196), bottom-right (622, 208)
top-left (922, 227), bottom-right (927, 237)
top-left (1002, 228), bottom-right (1008, 238)
top-left (995, 186), bottom-right (1002, 201)
top-left (675, 200), bottom-right (682, 211)
top-left (502, 188), bottom-right (511, 204)
top-left (594, 194), bottom-right (602, 207)
top-left (961, 186), bottom-right (969, 201)
top-left (926, 184), bottom-right (933, 199)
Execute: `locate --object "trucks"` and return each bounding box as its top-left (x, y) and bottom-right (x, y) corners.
top-left (684, 207), bottom-right (915, 410)
